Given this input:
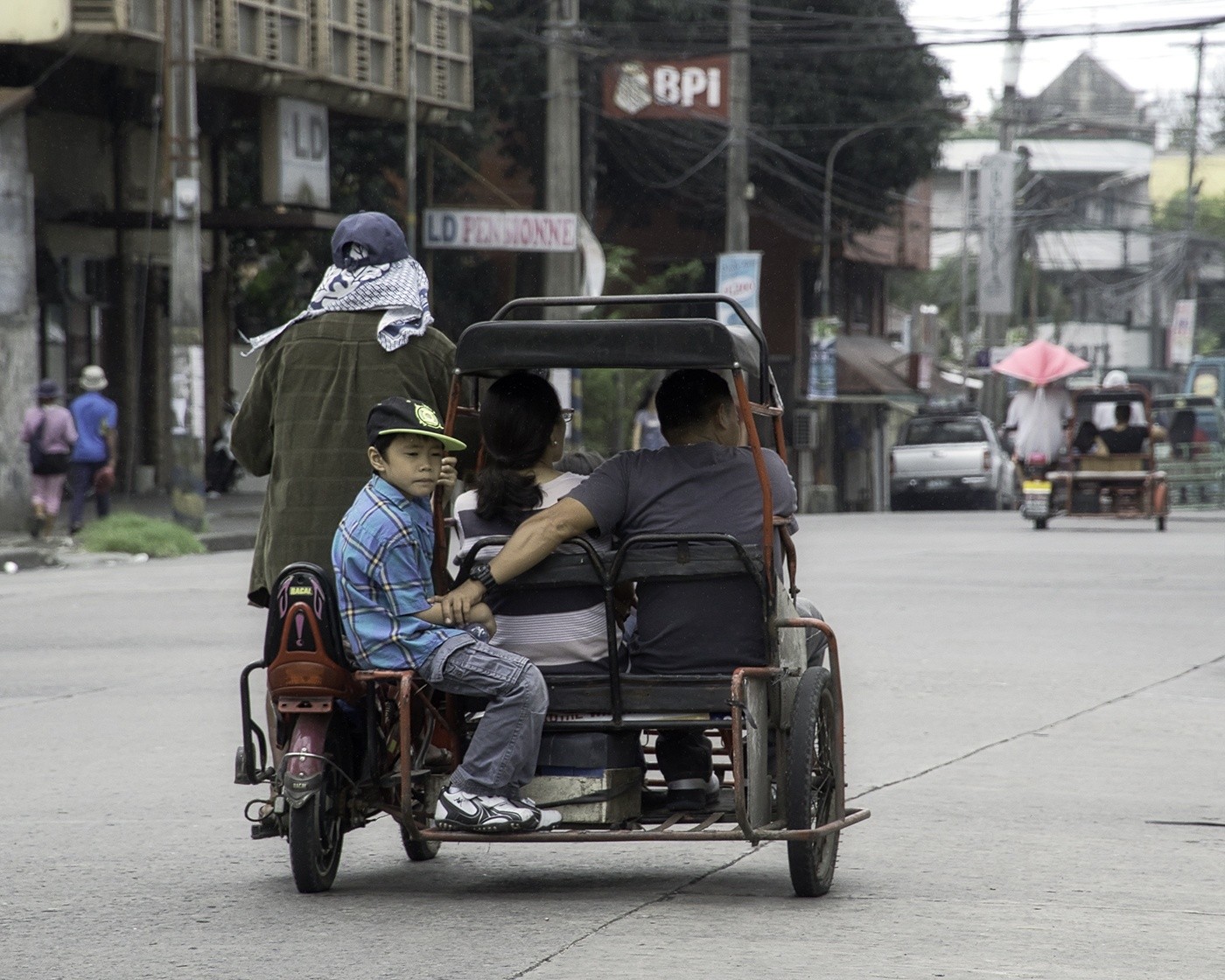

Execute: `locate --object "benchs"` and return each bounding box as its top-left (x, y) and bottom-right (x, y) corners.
top-left (1080, 453), bottom-right (1150, 471)
top-left (451, 530), bottom-right (769, 729)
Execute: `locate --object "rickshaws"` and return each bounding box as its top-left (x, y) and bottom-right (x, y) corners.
top-left (232, 290), bottom-right (873, 898)
top-left (1005, 354), bottom-right (1225, 533)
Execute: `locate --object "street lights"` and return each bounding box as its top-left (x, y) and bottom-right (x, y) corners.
top-left (811, 94), bottom-right (970, 490)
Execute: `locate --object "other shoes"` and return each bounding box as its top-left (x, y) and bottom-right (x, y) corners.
top-left (258, 806), bottom-right (274, 824)
top-left (32, 518), bottom-right (44, 537)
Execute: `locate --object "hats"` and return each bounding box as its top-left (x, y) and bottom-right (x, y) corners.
top-left (366, 396), bottom-right (467, 450)
top-left (78, 365), bottom-right (107, 391)
top-left (34, 380), bottom-right (63, 399)
top-left (331, 211), bottom-right (408, 271)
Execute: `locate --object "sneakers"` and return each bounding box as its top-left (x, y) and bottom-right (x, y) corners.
top-left (667, 770), bottom-right (720, 810)
top-left (508, 798), bottom-right (563, 831)
top-left (434, 785), bottom-right (541, 832)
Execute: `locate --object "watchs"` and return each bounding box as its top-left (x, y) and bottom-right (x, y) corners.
top-left (467, 561), bottom-right (499, 594)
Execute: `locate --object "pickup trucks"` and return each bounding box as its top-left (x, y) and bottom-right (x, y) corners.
top-left (884, 406), bottom-right (1020, 512)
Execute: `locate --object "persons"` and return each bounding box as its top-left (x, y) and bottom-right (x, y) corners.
top-left (427, 366), bottom-right (830, 807)
top-left (1168, 410), bottom-right (1210, 504)
top-left (1074, 401), bottom-right (1152, 456)
top-left (444, 371), bottom-right (633, 691)
top-left (1007, 373), bottom-right (1077, 515)
top-left (67, 366), bottom-right (120, 536)
top-left (229, 208), bottom-right (465, 602)
top-left (21, 380), bottom-right (78, 544)
top-left (333, 393), bottom-right (564, 835)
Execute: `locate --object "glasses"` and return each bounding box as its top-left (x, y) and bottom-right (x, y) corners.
top-left (561, 408), bottom-right (574, 422)
